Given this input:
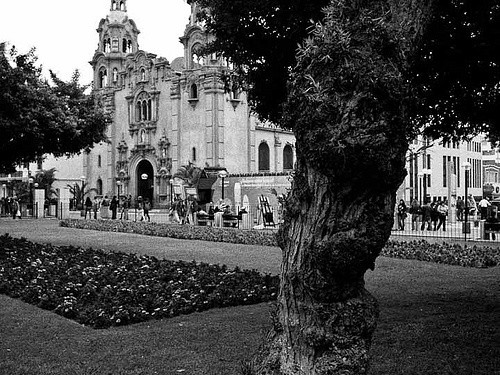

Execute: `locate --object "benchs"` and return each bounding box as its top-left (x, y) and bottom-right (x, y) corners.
top-left (485, 221), bottom-right (500, 241)
top-left (197, 214), bottom-right (242, 227)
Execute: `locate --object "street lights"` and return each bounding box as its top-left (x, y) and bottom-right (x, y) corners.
top-left (219, 170), bottom-right (227, 200)
top-left (80, 176), bottom-right (86, 209)
top-left (29, 178), bottom-right (33, 209)
top-left (72, 182), bottom-right (76, 207)
top-left (461, 161), bottom-right (471, 241)
top-left (2, 184), bottom-right (6, 198)
top-left (117, 180), bottom-right (121, 207)
top-left (141, 173), bottom-right (148, 199)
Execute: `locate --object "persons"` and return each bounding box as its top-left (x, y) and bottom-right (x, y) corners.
top-left (84, 195), bottom-right (151, 222)
top-left (44, 196), bottom-right (50, 217)
top-left (398, 187), bottom-right (500, 232)
top-left (169, 197), bottom-right (248, 228)
top-left (0, 196), bottom-right (22, 219)
